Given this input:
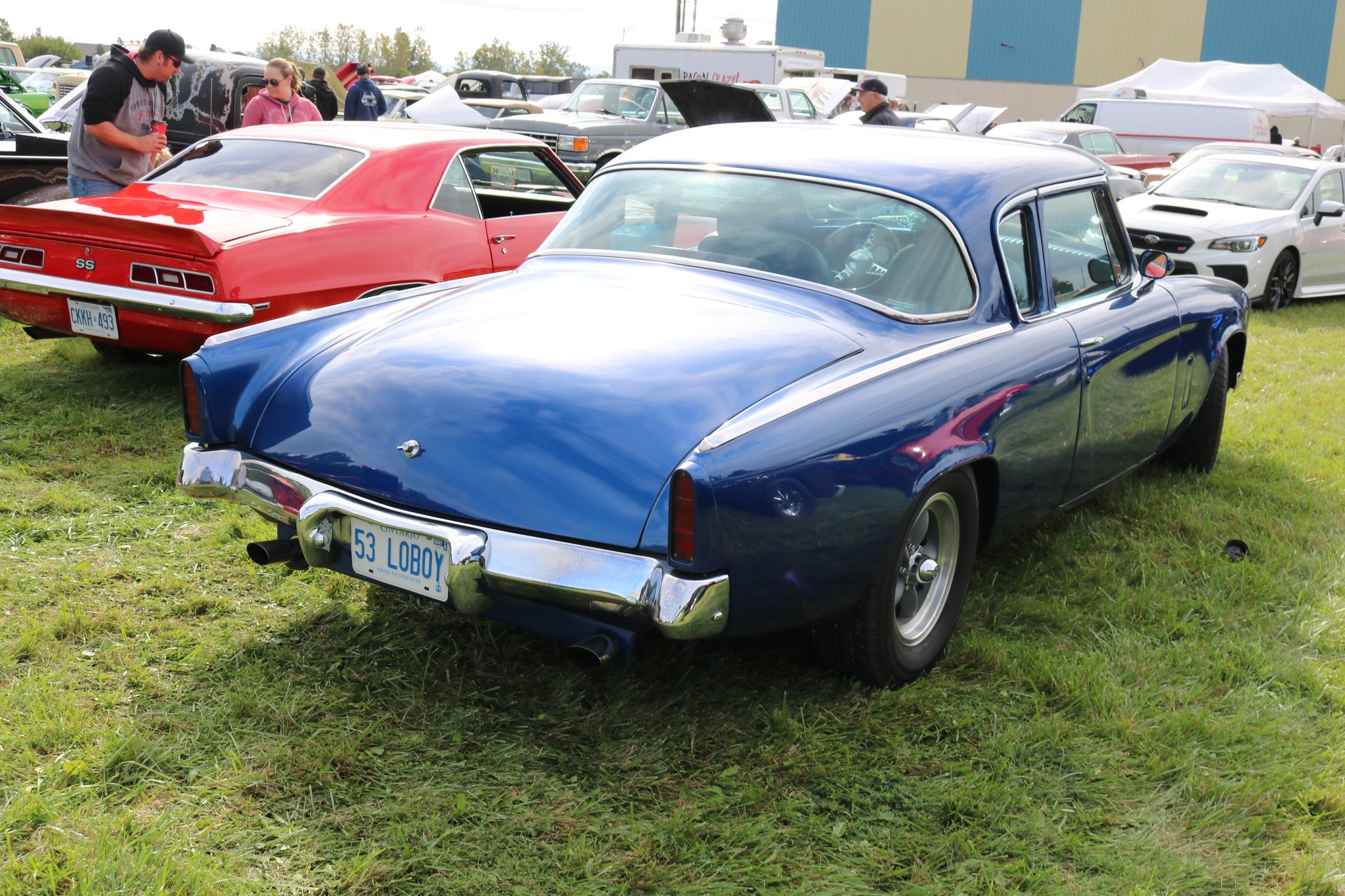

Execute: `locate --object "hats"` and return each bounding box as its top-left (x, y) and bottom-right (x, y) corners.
top-left (145, 29), bottom-right (196, 64)
top-left (355, 62), bottom-right (372, 75)
top-left (1294, 137), bottom-right (1301, 142)
top-left (850, 76), bottom-right (888, 96)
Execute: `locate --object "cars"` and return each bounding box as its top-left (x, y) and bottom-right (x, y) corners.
top-left (1080, 154), bottom-right (1345, 312)
top-left (477, 77), bottom-right (690, 187)
top-left (0, 41), bottom-right (650, 207)
top-left (656, 75), bottom-right (1344, 239)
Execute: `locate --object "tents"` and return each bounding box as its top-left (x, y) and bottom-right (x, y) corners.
top-left (1074, 57), bottom-right (1344, 150)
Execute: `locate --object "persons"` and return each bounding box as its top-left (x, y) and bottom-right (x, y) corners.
top-left (241, 58), bottom-right (338, 128)
top-left (1269, 123), bottom-right (1282, 145)
top-left (841, 96), bottom-right (852, 113)
top-left (68, 29), bottom-right (196, 198)
top-left (851, 78), bottom-right (907, 126)
top-left (1291, 136), bottom-right (1301, 147)
top-left (343, 63), bottom-right (387, 120)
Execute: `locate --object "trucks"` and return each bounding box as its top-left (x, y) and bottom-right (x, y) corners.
top-left (1055, 98), bottom-right (1272, 164)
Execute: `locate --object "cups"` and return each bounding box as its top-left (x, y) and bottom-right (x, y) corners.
top-left (150, 119), bottom-right (168, 151)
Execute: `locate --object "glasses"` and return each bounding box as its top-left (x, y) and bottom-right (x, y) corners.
top-left (152, 49), bottom-right (182, 68)
top-left (262, 77), bottom-right (287, 87)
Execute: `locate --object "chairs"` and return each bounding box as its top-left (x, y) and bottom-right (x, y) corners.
top-left (698, 200), bottom-right (839, 287)
top-left (433, 184), bottom-right (460, 214)
top-left (885, 219), bottom-right (1028, 308)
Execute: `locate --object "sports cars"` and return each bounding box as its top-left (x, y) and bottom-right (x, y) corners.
top-left (0, 119), bottom-right (719, 363)
top-left (181, 123), bottom-right (1250, 691)
